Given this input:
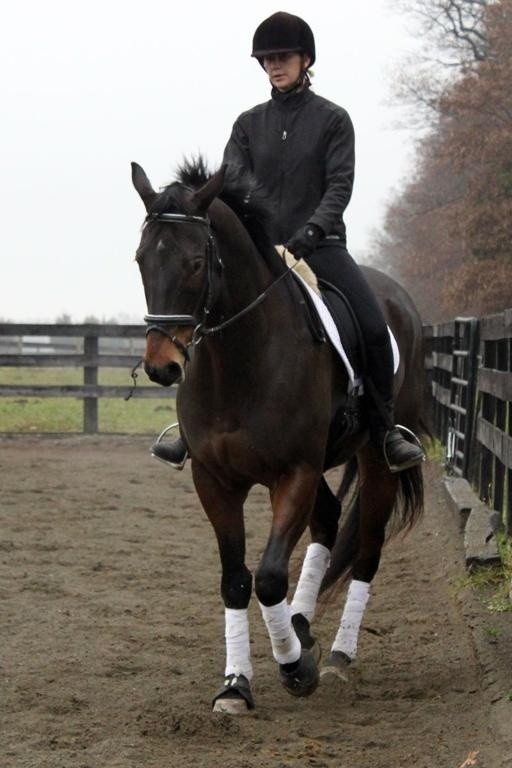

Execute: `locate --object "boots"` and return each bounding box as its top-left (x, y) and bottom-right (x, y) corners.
top-left (366, 397), bottom-right (423, 471)
top-left (149, 435), bottom-right (192, 463)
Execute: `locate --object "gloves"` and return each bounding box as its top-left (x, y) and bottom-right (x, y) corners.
top-left (283, 224), bottom-right (324, 261)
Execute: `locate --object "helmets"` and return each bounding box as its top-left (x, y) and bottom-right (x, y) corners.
top-left (250, 12), bottom-right (315, 69)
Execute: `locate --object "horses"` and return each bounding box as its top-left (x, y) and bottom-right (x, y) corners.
top-left (127, 151), bottom-right (432, 716)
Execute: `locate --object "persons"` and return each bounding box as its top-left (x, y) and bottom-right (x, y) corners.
top-left (151, 9), bottom-right (428, 471)
